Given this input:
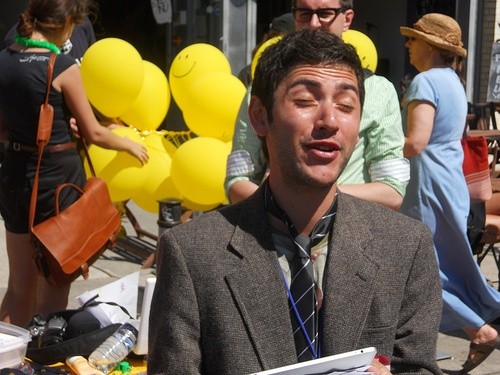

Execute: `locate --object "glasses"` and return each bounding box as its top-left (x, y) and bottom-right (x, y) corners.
top-left (292, 6), bottom-right (344, 24)
top-left (409, 37), bottom-right (416, 41)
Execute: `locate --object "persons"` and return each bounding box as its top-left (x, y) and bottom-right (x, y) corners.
top-left (147, 29), bottom-right (442, 375)
top-left (3, 15), bottom-right (97, 65)
top-left (400, 13), bottom-right (500, 374)
top-left (224, 0), bottom-right (410, 212)
top-left (0, 0), bottom-right (150, 328)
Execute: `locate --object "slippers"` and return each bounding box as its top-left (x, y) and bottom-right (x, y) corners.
top-left (460, 334), bottom-right (500, 374)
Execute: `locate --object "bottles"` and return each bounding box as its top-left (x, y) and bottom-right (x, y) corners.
top-left (88, 317), bottom-right (141, 375)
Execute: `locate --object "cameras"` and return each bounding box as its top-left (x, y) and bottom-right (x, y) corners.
top-left (32, 317), bottom-right (68, 348)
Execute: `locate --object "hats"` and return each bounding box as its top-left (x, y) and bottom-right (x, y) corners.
top-left (270, 13), bottom-right (294, 34)
top-left (400, 13), bottom-right (467, 57)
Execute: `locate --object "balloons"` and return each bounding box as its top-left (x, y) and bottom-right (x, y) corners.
top-left (79, 30), bottom-right (290, 214)
top-left (341, 29), bottom-right (378, 73)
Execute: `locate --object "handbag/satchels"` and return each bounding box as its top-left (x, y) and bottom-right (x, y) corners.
top-left (461, 130), bottom-right (492, 201)
top-left (31, 178), bottom-right (121, 288)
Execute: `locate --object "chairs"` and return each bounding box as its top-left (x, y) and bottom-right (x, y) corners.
top-left (466, 101), bottom-right (500, 274)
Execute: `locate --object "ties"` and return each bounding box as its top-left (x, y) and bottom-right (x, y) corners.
top-left (265, 186), bottom-right (338, 362)
